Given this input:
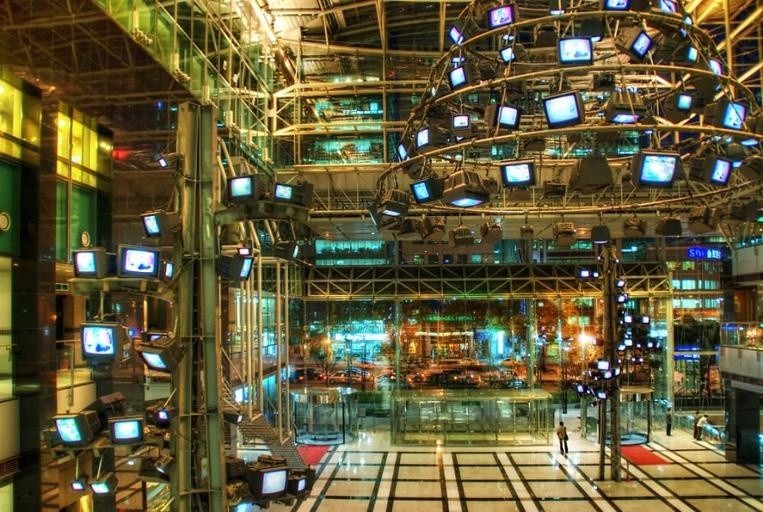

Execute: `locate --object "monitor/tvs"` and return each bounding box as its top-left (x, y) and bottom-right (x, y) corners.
top-left (575, 270), bottom-right (661, 408)
top-left (368, 0), bottom-right (763, 247)
top-left (51, 174), bottom-right (308, 499)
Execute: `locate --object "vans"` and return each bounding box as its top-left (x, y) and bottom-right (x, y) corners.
top-left (348, 393), bottom-right (389, 417)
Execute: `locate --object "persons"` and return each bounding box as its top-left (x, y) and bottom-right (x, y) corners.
top-left (556, 422), bottom-right (569, 458)
top-left (696, 415), bottom-right (708, 440)
top-left (666, 408), bottom-right (672, 436)
top-left (693, 411), bottom-right (700, 439)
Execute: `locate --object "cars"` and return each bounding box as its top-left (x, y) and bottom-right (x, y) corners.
top-left (561, 378), bottom-right (596, 394)
top-left (399, 357), bottom-right (527, 389)
top-left (282, 364), bottom-right (369, 385)
